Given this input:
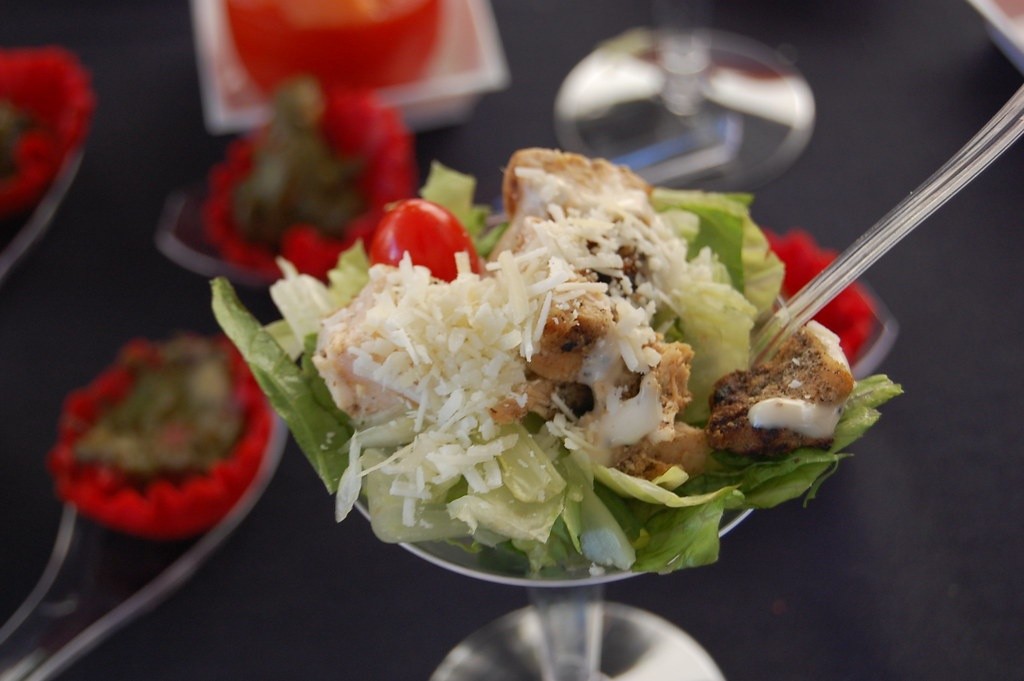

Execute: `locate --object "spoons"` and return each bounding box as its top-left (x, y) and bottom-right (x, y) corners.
top-left (0, 406), bottom-right (291, 681)
top-left (0, 146), bottom-right (83, 283)
top-left (152, 114), bottom-right (743, 291)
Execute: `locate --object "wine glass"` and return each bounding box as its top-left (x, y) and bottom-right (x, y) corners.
top-left (351, 500), bottom-right (754, 681)
top-left (555, 1), bottom-right (816, 195)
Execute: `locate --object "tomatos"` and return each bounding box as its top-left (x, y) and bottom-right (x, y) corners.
top-left (372, 198), bottom-right (479, 283)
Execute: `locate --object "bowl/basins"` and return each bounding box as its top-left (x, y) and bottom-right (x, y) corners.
top-left (188, 0), bottom-right (513, 154)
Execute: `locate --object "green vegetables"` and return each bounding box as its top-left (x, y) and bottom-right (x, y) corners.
top-left (211, 161), bottom-right (902, 572)
